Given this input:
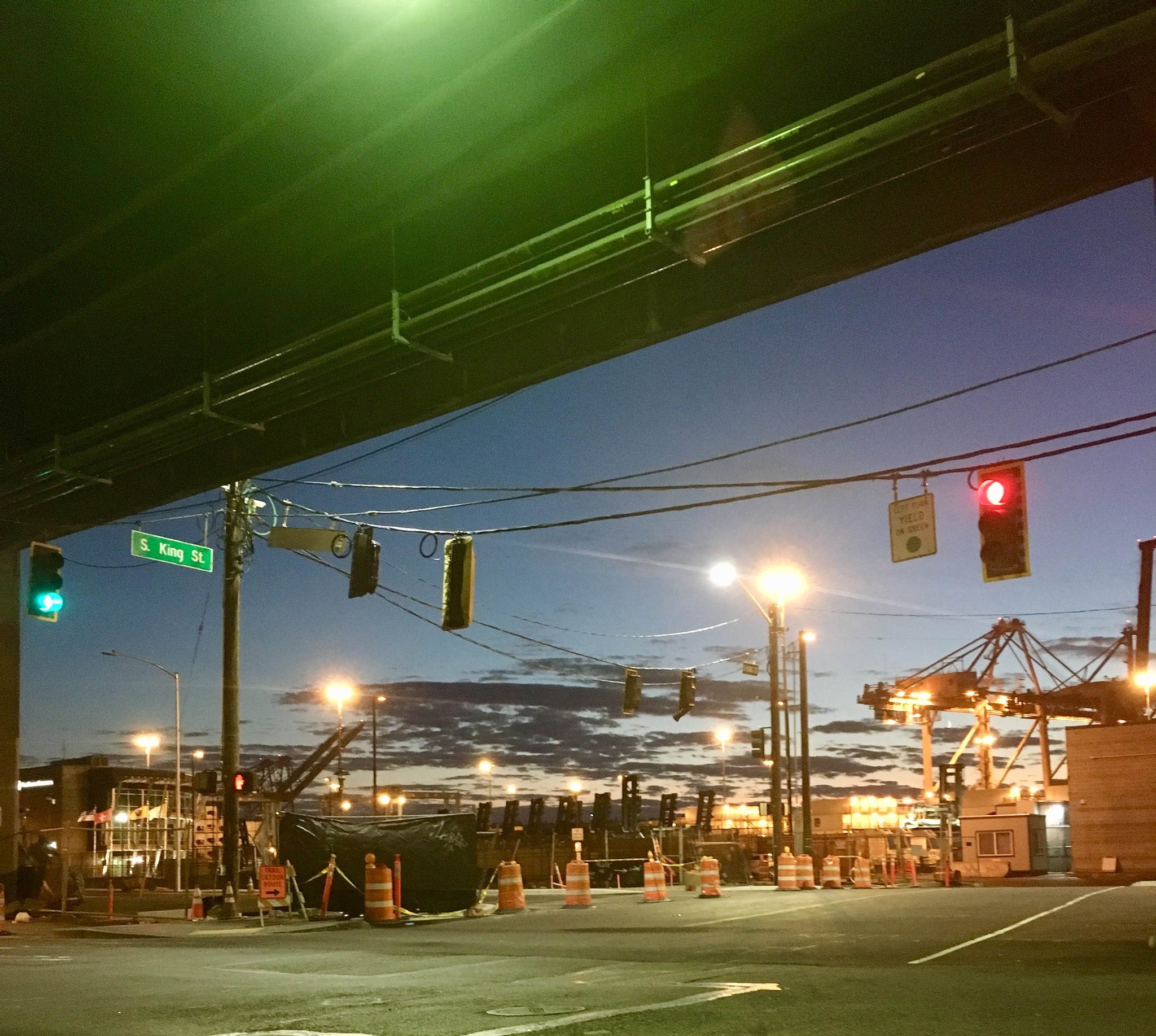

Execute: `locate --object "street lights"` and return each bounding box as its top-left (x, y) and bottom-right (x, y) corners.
top-left (792, 630), bottom-right (814, 864)
top-left (328, 681), bottom-right (349, 802)
top-left (372, 695), bottom-right (387, 813)
top-left (100, 650), bottom-right (181, 892)
top-left (479, 760), bottom-right (492, 802)
top-left (707, 559), bottom-right (786, 884)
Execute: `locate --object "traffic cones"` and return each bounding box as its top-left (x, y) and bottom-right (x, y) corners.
top-left (218, 881), bottom-right (241, 920)
top-left (188, 884), bottom-right (205, 921)
top-left (247, 875), bottom-right (254, 891)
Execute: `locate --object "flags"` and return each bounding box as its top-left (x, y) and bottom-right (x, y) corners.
top-left (95, 808), bottom-right (111, 823)
top-left (129, 805), bottom-right (149, 820)
top-left (78, 810), bottom-right (95, 822)
top-left (149, 803), bottom-right (166, 821)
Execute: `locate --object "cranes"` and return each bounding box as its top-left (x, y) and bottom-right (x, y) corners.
top-left (856, 613), bottom-right (1156, 820)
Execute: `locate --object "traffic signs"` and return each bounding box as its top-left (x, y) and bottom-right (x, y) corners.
top-left (260, 865), bottom-right (286, 899)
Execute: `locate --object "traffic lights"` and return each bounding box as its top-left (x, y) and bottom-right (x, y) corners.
top-left (26, 541), bottom-right (65, 623)
top-left (234, 772), bottom-right (251, 791)
top-left (974, 460), bottom-right (1032, 582)
top-left (348, 526), bottom-right (381, 599)
top-left (679, 672), bottom-right (697, 711)
top-left (623, 669), bottom-right (642, 715)
